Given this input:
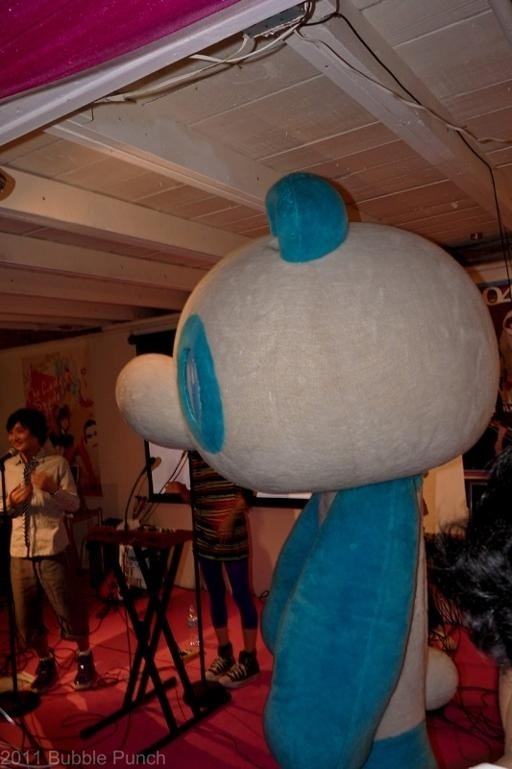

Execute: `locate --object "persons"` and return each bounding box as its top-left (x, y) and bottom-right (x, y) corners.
top-left (163, 449), bottom-right (262, 689)
top-left (0, 405), bottom-right (100, 695)
top-left (83, 419), bottom-right (98, 461)
top-left (44, 404), bottom-right (75, 448)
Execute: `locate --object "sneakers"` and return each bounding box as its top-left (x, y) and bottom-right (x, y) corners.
top-left (204, 655), bottom-right (263, 690)
top-left (70, 649), bottom-right (97, 690)
top-left (29, 657), bottom-right (59, 693)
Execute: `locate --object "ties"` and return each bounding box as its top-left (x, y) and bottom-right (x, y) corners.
top-left (23, 460), bottom-right (40, 548)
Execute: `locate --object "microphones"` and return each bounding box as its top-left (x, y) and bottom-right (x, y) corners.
top-left (124, 457), bottom-right (156, 530)
top-left (0, 448), bottom-right (17, 463)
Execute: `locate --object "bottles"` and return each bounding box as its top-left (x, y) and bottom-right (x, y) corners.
top-left (186, 605), bottom-right (200, 648)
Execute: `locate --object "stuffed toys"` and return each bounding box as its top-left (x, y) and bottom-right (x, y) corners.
top-left (115, 170), bottom-right (503, 769)
